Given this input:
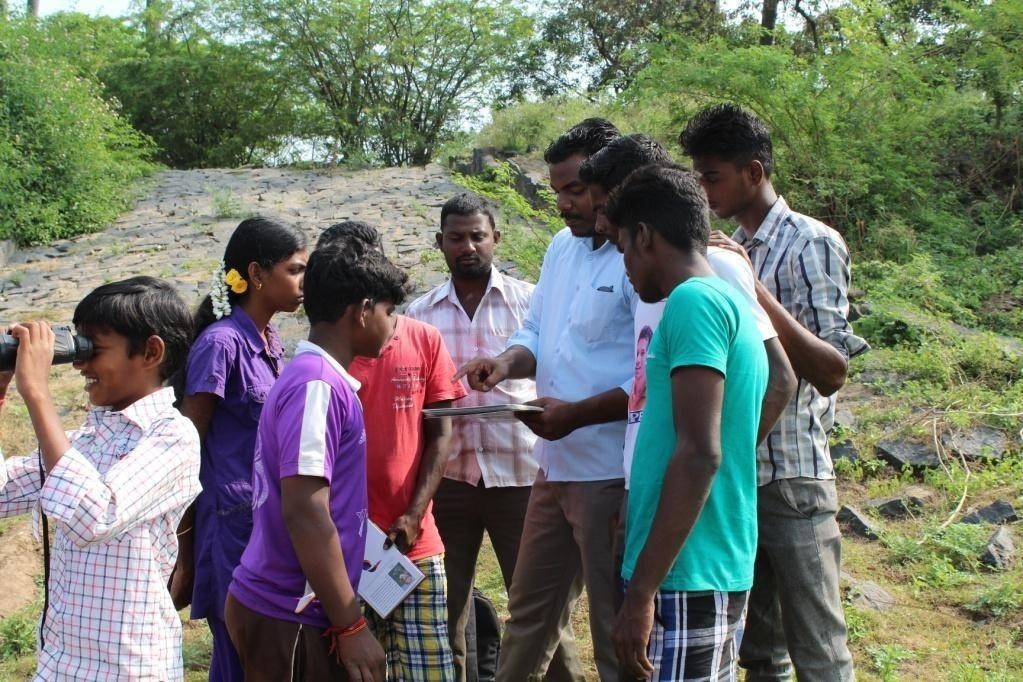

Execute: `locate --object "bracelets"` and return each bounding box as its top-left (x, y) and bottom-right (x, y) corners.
top-left (320, 615), bottom-right (367, 663)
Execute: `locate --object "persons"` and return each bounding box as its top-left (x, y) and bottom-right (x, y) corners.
top-left (223, 236), bottom-right (410, 682)
top-left (0, 275), bottom-right (203, 682)
top-left (402, 189), bottom-right (586, 682)
top-left (449, 101), bottom-right (872, 682)
top-left (315, 222), bottom-right (468, 682)
top-left (170, 216), bottom-right (309, 682)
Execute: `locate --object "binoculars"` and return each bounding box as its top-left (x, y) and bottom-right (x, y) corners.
top-left (0, 324), bottom-right (94, 371)
